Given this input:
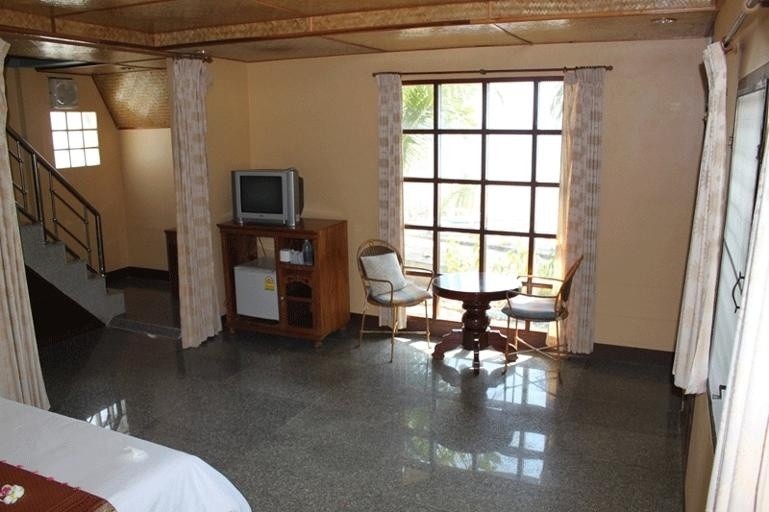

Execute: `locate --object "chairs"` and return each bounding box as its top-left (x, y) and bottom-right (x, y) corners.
top-left (356, 239), bottom-right (434, 363)
top-left (500, 254), bottom-right (585, 382)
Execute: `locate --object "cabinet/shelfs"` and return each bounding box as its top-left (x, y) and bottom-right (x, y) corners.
top-left (216, 218), bottom-right (352, 347)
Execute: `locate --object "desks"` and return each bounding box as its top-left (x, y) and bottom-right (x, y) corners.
top-left (0, 392), bottom-right (252, 512)
top-left (432, 270), bottom-right (524, 376)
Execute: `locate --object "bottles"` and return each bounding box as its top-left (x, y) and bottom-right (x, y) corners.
top-left (303, 239), bottom-right (313, 266)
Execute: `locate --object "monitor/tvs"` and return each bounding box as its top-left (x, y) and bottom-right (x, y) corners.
top-left (232, 167), bottom-right (306, 227)
